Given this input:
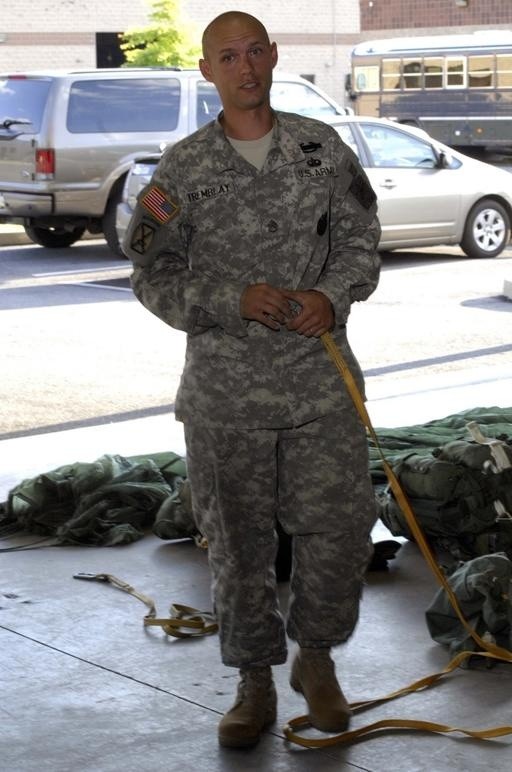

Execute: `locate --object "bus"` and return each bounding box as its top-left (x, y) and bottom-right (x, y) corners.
top-left (342, 26), bottom-right (512, 155)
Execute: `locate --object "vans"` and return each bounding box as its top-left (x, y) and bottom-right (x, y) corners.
top-left (1, 68), bottom-right (351, 266)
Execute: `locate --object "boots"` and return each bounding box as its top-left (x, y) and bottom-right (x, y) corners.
top-left (289, 644), bottom-right (354, 735)
top-left (217, 664), bottom-right (277, 751)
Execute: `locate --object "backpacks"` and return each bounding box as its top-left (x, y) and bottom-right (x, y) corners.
top-left (0, 451), bottom-right (209, 552)
top-left (425, 551), bottom-right (512, 670)
top-left (376, 420), bottom-right (512, 544)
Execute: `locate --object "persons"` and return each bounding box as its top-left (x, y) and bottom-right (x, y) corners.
top-left (120, 11), bottom-right (381, 750)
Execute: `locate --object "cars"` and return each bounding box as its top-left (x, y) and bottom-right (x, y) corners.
top-left (299, 110), bottom-right (512, 256)
